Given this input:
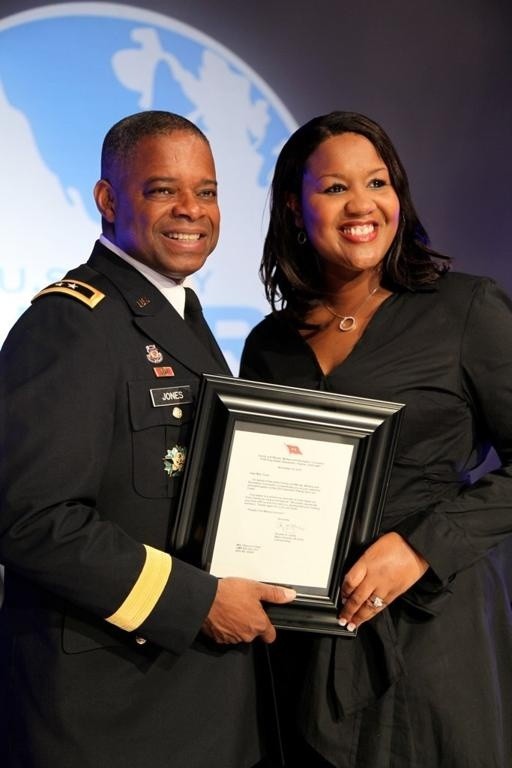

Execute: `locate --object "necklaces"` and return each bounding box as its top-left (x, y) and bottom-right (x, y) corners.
top-left (319, 283), bottom-right (381, 333)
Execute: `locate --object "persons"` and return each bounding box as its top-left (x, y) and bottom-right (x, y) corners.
top-left (0, 110), bottom-right (302, 767)
top-left (237, 110), bottom-right (512, 768)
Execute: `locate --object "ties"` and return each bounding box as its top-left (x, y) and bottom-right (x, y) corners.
top-left (184, 287), bottom-right (233, 376)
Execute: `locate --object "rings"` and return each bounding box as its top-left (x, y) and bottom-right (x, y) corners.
top-left (368, 594), bottom-right (386, 608)
